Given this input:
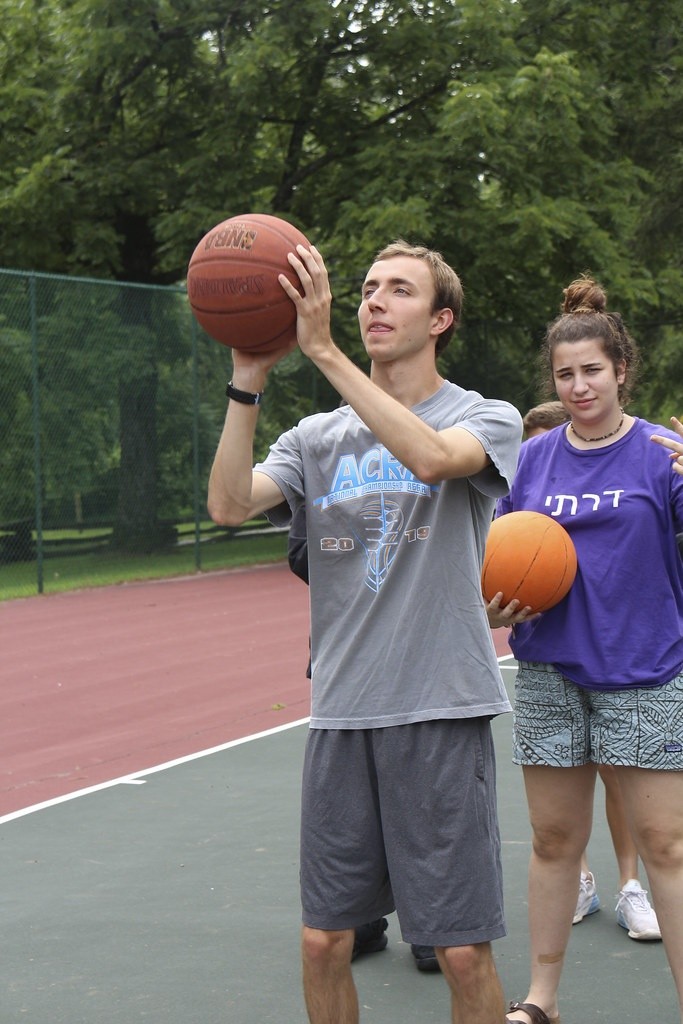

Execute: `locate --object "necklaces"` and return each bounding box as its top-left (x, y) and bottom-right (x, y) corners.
top-left (570, 406), bottom-right (625, 442)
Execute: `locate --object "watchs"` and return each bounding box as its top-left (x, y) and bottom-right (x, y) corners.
top-left (224, 379), bottom-right (264, 405)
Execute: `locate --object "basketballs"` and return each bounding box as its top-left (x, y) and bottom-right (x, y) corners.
top-left (186, 213), bottom-right (311, 354)
top-left (481, 510), bottom-right (579, 616)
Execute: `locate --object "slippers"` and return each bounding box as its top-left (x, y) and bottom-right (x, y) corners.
top-left (505, 1000), bottom-right (560, 1024)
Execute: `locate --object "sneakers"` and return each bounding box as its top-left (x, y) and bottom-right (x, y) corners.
top-left (615, 889), bottom-right (662, 940)
top-left (350, 931), bottom-right (389, 963)
top-left (410, 942), bottom-right (442, 972)
top-left (572, 872), bottom-right (600, 924)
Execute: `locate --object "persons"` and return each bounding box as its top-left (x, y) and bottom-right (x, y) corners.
top-left (288, 518), bottom-right (448, 974)
top-left (202, 238), bottom-right (524, 1024)
top-left (502, 283), bottom-right (683, 1024)
top-left (522, 402), bottom-right (664, 943)
